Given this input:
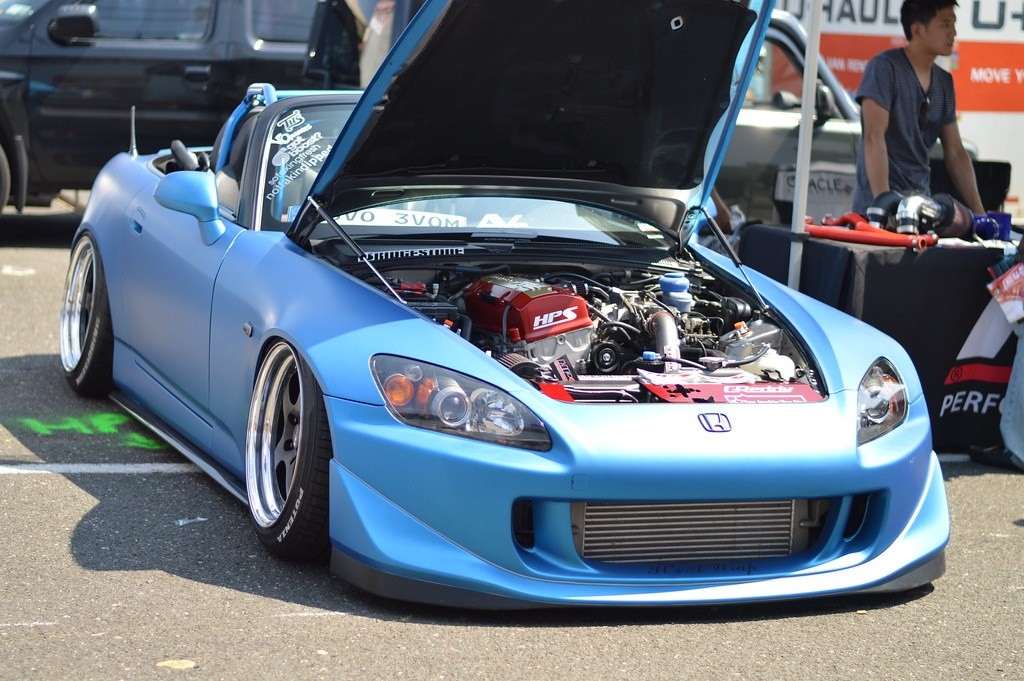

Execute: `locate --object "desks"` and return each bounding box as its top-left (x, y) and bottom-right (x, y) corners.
top-left (738, 222), bottom-right (1024, 447)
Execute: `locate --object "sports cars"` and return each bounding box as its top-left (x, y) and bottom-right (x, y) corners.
top-left (59, 1), bottom-right (953, 615)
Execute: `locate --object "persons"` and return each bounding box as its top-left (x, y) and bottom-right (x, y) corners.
top-left (849, 0), bottom-right (986, 231)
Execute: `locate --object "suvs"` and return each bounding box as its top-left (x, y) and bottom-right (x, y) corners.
top-left (1, 1), bottom-right (417, 228)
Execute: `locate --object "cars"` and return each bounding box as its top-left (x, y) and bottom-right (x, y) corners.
top-left (708, 5), bottom-right (978, 243)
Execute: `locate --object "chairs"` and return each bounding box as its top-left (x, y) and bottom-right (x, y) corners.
top-left (217, 111), bottom-right (319, 232)
